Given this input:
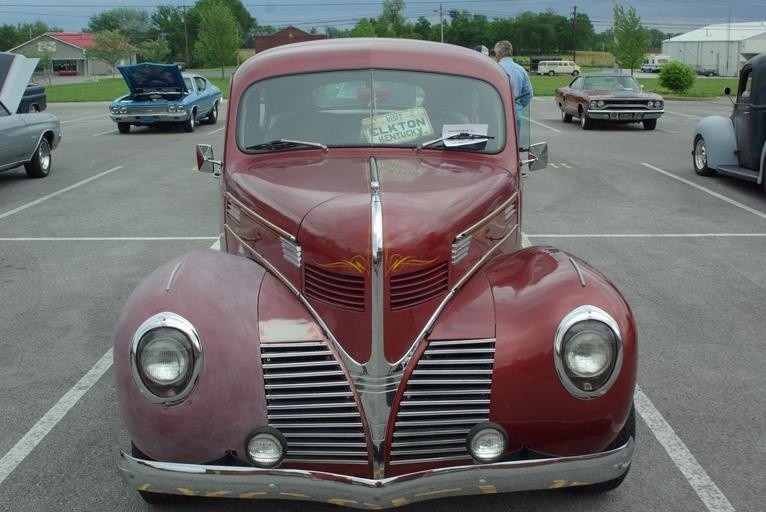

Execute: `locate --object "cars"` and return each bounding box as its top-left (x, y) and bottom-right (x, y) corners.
top-left (553, 71), bottom-right (665, 131)
top-left (691, 65), bottom-right (716, 77)
top-left (108, 62), bottom-right (222, 134)
top-left (0, 50), bottom-right (63, 182)
top-left (113, 36), bottom-right (638, 512)
top-left (690, 52), bottom-right (766, 189)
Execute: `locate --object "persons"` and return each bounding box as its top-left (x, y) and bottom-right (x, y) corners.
top-left (487, 40), bottom-right (535, 179)
top-left (473, 45), bottom-right (490, 55)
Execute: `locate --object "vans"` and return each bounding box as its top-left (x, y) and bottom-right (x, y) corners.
top-left (537, 60), bottom-right (582, 76)
top-left (640, 53), bottom-right (672, 73)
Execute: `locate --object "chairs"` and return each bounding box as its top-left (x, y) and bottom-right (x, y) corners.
top-left (264, 112), bottom-right (319, 147)
top-left (420, 111), bottom-right (470, 144)
